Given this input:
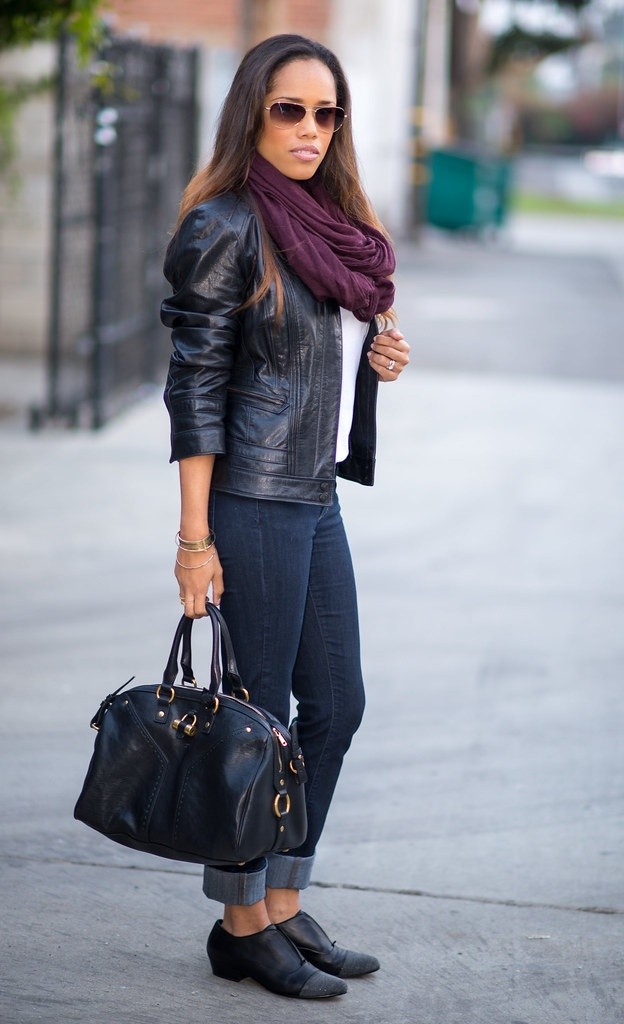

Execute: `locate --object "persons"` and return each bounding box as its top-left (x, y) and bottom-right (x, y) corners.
top-left (159, 33), bottom-right (411, 1001)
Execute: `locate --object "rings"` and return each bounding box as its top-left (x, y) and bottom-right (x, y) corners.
top-left (387, 359), bottom-right (395, 370)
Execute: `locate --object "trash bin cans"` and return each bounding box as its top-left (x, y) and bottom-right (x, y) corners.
top-left (420, 146), bottom-right (505, 234)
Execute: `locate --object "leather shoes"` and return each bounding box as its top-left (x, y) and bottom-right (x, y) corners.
top-left (206, 919), bottom-right (348, 999)
top-left (277, 909), bottom-right (380, 977)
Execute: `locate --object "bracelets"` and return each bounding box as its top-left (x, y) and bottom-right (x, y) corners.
top-left (174, 528), bottom-right (216, 569)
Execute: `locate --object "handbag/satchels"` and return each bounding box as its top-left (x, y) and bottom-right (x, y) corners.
top-left (72, 603), bottom-right (308, 863)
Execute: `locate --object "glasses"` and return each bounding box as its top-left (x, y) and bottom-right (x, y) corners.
top-left (262, 102), bottom-right (349, 134)
